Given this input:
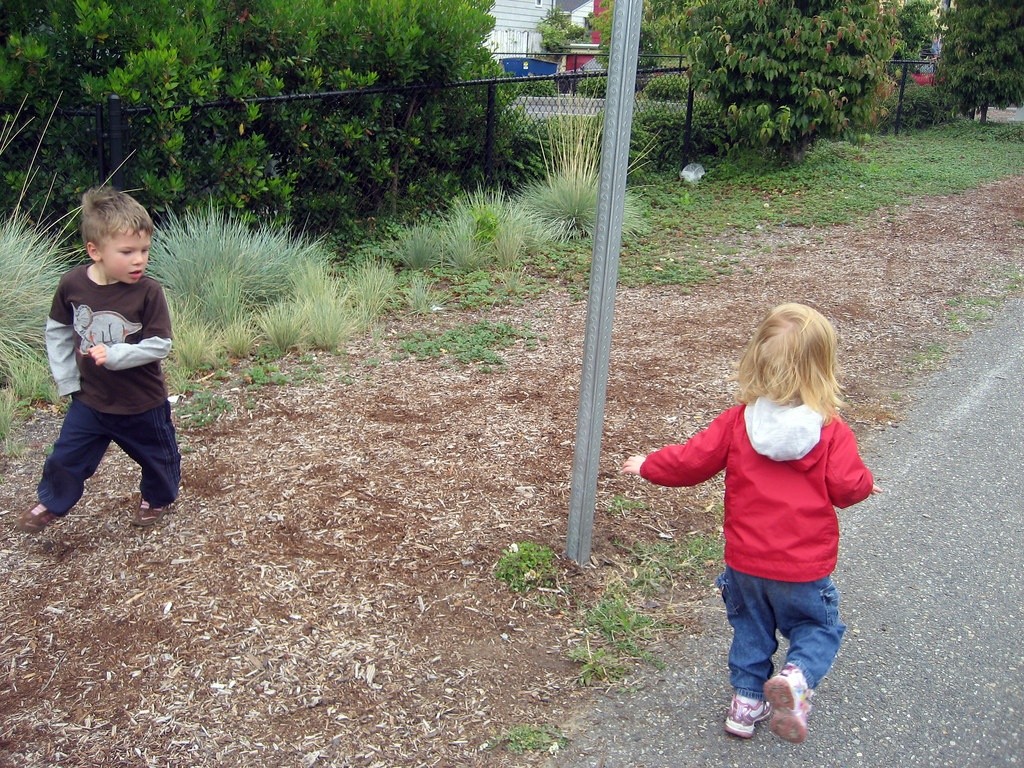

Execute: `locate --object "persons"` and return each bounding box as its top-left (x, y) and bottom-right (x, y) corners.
top-left (623, 302), bottom-right (884, 743)
top-left (14, 187), bottom-right (181, 532)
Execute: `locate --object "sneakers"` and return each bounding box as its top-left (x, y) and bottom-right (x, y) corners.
top-left (132, 496), bottom-right (168, 526)
top-left (763, 662), bottom-right (814, 743)
top-left (724, 694), bottom-right (771, 738)
top-left (14, 502), bottom-right (57, 533)
